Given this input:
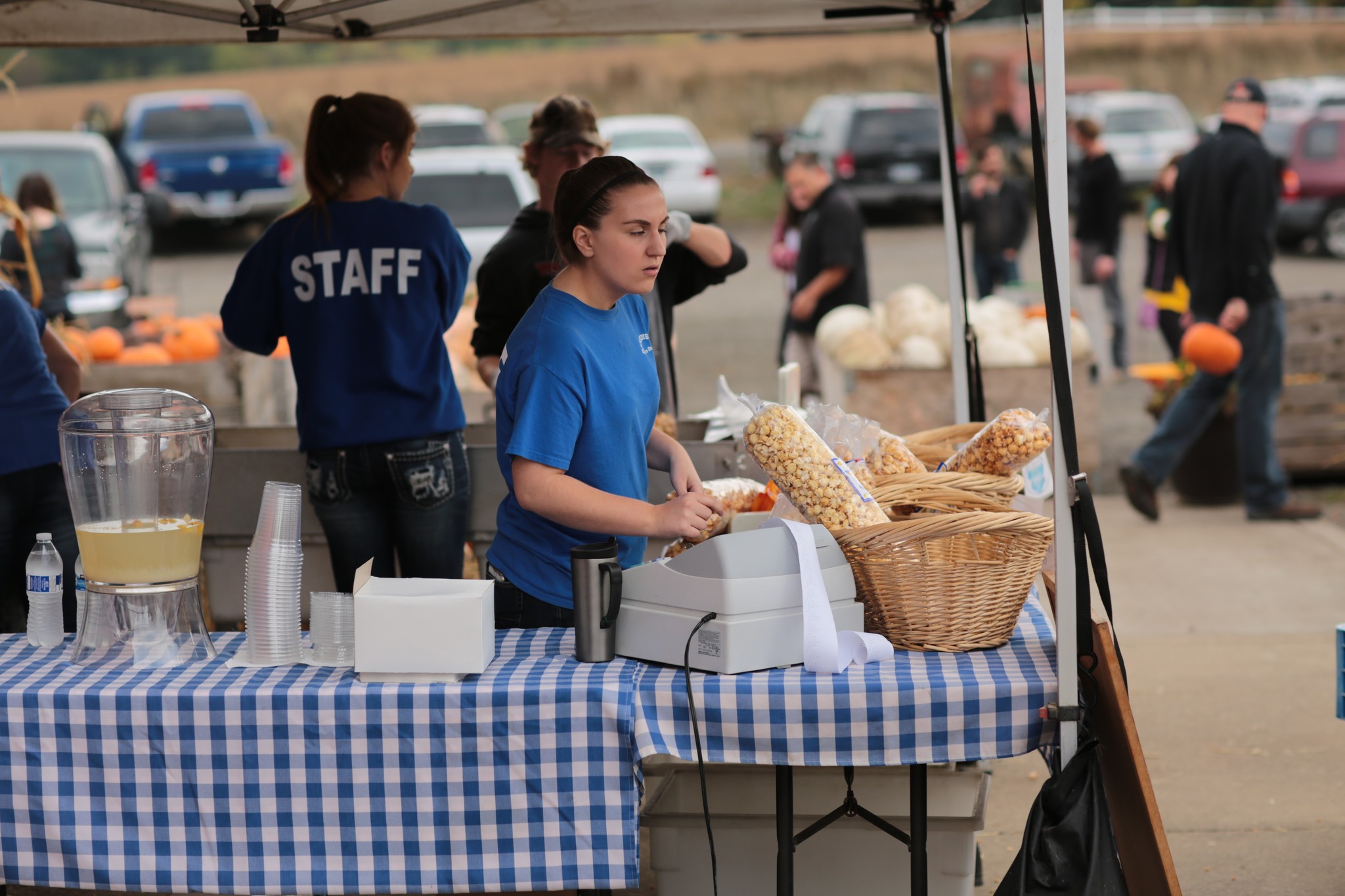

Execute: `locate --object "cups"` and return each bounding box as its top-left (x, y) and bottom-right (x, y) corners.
top-left (243, 480), bottom-right (304, 666)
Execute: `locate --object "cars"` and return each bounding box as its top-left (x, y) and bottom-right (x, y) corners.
top-left (0, 129), bottom-right (154, 340)
top-left (396, 100), bottom-right (721, 284)
top-left (1061, 88), bottom-right (1201, 186)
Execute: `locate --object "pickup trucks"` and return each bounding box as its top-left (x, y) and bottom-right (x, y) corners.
top-left (118, 88), bottom-right (297, 227)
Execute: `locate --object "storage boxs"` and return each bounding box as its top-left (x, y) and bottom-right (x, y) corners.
top-left (808, 335), bottom-right (1101, 492)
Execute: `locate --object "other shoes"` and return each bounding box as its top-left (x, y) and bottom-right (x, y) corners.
top-left (1119, 466), bottom-right (1161, 519)
top-left (1246, 500), bottom-right (1316, 520)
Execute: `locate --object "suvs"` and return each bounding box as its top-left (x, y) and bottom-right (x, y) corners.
top-left (780, 93), bottom-right (978, 227)
top-left (1201, 76), bottom-right (1344, 259)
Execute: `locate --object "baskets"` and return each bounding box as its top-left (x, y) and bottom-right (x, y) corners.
top-left (832, 484), bottom-right (1053, 649)
top-left (866, 422), bottom-right (1026, 520)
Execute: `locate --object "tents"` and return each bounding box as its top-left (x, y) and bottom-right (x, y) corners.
top-left (0, 0), bottom-right (1079, 769)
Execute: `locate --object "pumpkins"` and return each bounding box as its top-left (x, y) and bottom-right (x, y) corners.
top-left (52, 310), bottom-right (226, 367)
top-left (813, 283), bottom-right (1088, 371)
top-left (1180, 321), bottom-right (1243, 376)
top-left (1125, 358), bottom-right (1194, 380)
top-left (270, 336), bottom-right (290, 360)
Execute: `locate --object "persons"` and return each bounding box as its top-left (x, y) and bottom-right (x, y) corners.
top-left (485, 155), bottom-right (724, 629)
top-left (1144, 156), bottom-right (1190, 358)
top-left (0, 284), bottom-right (99, 634)
top-left (221, 92), bottom-right (476, 592)
top-left (469, 94), bottom-right (749, 417)
top-left (1068, 119), bottom-right (1125, 371)
top-left (772, 154), bottom-right (869, 399)
top-left (960, 99), bottom-right (1031, 297)
top-left (0, 175), bottom-right (81, 324)
top-left (1118, 79), bottom-right (1323, 521)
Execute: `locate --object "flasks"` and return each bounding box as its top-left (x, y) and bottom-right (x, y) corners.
top-left (570, 536), bottom-right (622, 662)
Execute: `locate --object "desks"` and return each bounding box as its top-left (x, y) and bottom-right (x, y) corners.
top-left (0, 574), bottom-right (1060, 896)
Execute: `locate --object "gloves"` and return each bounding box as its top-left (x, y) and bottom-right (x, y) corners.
top-left (664, 210), bottom-right (691, 245)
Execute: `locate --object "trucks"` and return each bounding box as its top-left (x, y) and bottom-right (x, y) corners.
top-left (956, 45), bottom-right (1127, 145)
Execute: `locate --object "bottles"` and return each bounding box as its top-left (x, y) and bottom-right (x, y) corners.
top-left (25, 532), bottom-right (65, 648)
top-left (75, 553), bottom-right (117, 646)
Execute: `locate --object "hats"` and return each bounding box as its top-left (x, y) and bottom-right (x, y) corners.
top-left (530, 95), bottom-right (606, 147)
top-left (1223, 78), bottom-right (1264, 102)
